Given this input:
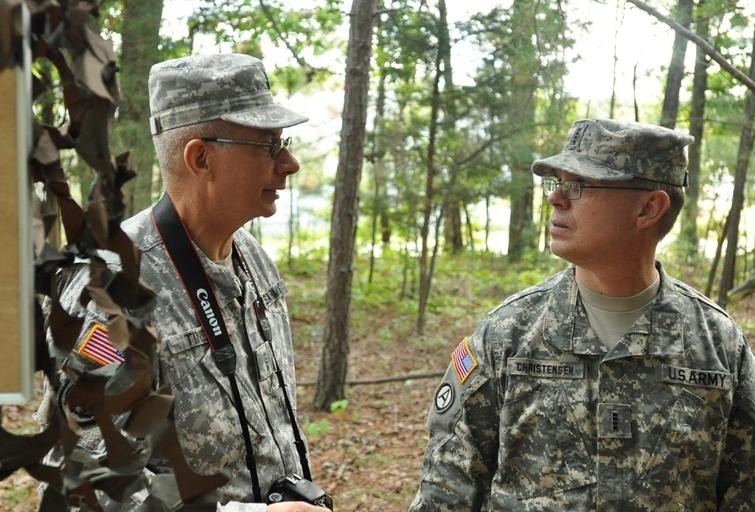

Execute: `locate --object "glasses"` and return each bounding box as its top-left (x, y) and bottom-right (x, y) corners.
top-left (541, 177), bottom-right (657, 201)
top-left (202, 135), bottom-right (294, 161)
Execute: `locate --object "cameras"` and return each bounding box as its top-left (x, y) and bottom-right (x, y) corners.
top-left (266, 473), bottom-right (334, 511)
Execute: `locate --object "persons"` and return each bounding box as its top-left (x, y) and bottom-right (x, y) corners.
top-left (33, 53), bottom-right (333, 511)
top-left (407, 119), bottom-right (754, 511)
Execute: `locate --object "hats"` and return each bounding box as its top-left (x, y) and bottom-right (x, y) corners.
top-left (145, 51), bottom-right (310, 136)
top-left (530, 117), bottom-right (696, 189)
